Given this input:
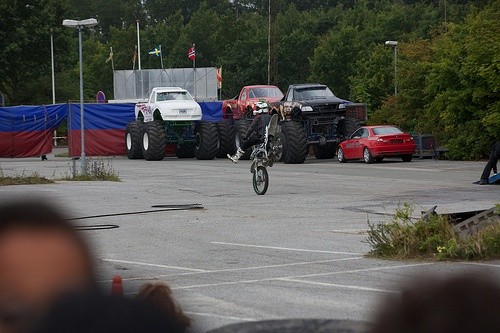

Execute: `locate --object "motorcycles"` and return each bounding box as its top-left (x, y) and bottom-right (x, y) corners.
top-left (249, 113), bottom-right (288, 196)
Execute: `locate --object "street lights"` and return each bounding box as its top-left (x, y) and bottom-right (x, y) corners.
top-left (385, 40), bottom-right (398, 117)
top-left (62, 18), bottom-right (98, 180)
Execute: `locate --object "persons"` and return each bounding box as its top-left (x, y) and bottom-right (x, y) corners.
top-left (227, 102), bottom-right (271, 163)
top-left (0, 200), bottom-right (192, 333)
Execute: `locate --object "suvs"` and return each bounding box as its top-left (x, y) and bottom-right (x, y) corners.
top-left (266, 84), bottom-right (355, 164)
top-left (216, 84), bottom-right (287, 161)
top-left (125, 86), bottom-right (219, 161)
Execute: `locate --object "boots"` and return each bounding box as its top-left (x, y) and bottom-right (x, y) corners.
top-left (227, 147), bottom-right (246, 163)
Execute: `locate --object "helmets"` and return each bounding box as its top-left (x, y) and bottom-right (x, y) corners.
top-left (253, 102), bottom-right (269, 115)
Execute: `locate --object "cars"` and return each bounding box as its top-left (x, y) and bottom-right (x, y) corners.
top-left (336, 124), bottom-right (416, 162)
